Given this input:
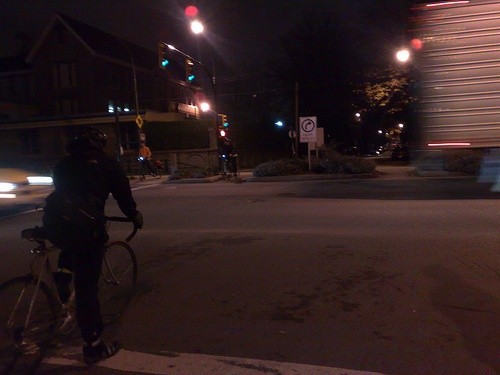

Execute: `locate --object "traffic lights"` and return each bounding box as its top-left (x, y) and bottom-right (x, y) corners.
top-left (220, 130), bottom-right (225, 137)
top-left (217, 114), bottom-right (228, 129)
top-left (185, 59), bottom-right (194, 84)
top-left (158, 42), bottom-right (168, 70)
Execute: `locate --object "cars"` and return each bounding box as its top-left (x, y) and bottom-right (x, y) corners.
top-left (390, 145), bottom-right (410, 162)
top-left (341, 146), bottom-right (368, 158)
top-left (0, 166), bottom-right (54, 216)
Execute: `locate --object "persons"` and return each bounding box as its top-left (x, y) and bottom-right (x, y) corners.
top-left (138, 141), bottom-right (157, 181)
top-left (219, 140), bottom-right (238, 177)
top-left (41, 126), bottom-right (144, 365)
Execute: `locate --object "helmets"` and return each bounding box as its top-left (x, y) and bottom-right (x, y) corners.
top-left (68, 125), bottom-right (107, 149)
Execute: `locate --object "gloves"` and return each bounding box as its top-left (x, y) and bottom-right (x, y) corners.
top-left (131, 212), bottom-right (143, 228)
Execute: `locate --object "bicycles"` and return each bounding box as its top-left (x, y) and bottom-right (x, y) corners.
top-left (136, 156), bottom-right (161, 182)
top-left (0, 216), bottom-right (143, 375)
top-left (219, 151), bottom-right (239, 182)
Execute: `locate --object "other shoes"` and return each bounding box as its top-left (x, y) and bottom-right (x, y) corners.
top-left (83, 341), bottom-right (120, 366)
top-left (53, 271), bottom-right (72, 285)
